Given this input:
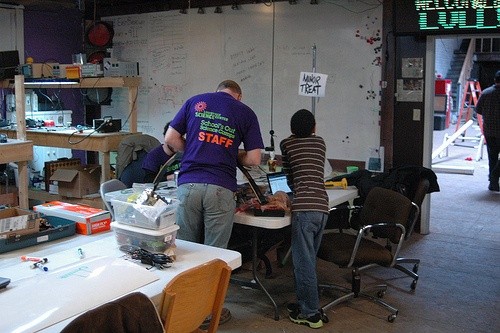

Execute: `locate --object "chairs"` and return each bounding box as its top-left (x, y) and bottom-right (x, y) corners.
top-left (317, 171), bottom-right (430, 324)
top-left (100, 179), bottom-right (127, 218)
top-left (160, 259), bottom-right (232, 333)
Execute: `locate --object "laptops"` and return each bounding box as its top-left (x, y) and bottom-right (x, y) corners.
top-left (266, 171), bottom-right (295, 196)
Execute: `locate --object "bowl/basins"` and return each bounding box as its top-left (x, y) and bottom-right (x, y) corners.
top-left (110, 191), bottom-right (181, 255)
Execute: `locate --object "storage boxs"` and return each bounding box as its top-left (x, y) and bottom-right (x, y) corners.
top-left (0, 164), bottom-right (110, 252)
top-left (105, 188), bottom-right (180, 254)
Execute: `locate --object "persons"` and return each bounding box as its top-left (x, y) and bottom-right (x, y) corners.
top-left (142, 120), bottom-right (171, 183)
top-left (476, 70), bottom-right (500, 192)
top-left (166, 80), bottom-right (265, 326)
top-left (279, 109), bottom-right (329, 327)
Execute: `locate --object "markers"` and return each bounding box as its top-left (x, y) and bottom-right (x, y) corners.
top-left (32, 258), bottom-right (48, 269)
top-left (20, 256), bottom-right (40, 261)
top-left (78, 248), bottom-right (83, 259)
top-left (37, 263), bottom-right (48, 271)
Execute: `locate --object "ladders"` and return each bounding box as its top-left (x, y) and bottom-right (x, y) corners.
top-left (452, 78), bottom-right (487, 145)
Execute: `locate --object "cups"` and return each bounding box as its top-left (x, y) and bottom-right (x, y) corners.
top-left (268, 160), bottom-right (277, 173)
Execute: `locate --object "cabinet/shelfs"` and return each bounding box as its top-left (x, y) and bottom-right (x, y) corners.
top-left (0, 77), bottom-right (142, 210)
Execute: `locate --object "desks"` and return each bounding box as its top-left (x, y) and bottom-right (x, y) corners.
top-left (0, 230), bottom-right (242, 333)
top-left (105, 166), bottom-right (358, 321)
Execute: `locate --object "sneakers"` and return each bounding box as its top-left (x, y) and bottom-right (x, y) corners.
top-left (286, 301), bottom-right (301, 313)
top-left (289, 310), bottom-right (323, 328)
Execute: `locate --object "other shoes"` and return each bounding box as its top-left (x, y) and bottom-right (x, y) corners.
top-left (199, 308), bottom-right (232, 330)
